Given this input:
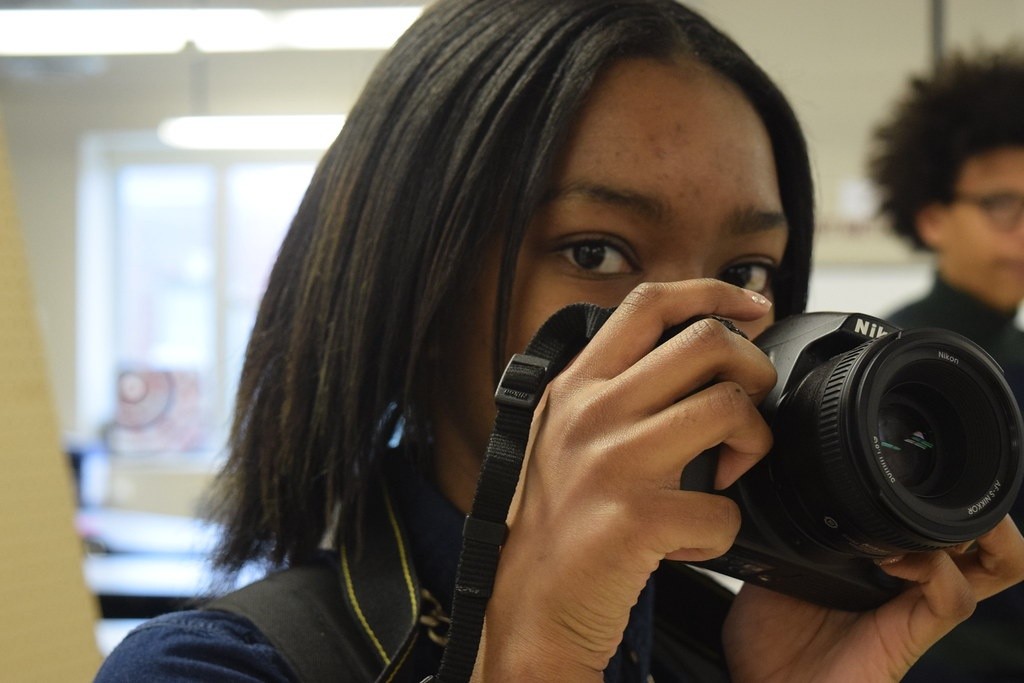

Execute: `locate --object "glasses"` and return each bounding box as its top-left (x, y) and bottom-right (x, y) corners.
top-left (953, 192), bottom-right (1024, 233)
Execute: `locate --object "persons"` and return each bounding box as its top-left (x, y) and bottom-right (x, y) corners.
top-left (94, 0), bottom-right (1024, 683)
top-left (862, 24), bottom-right (1024, 683)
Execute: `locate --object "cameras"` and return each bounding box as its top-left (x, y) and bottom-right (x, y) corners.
top-left (637, 313), bottom-right (1024, 614)
